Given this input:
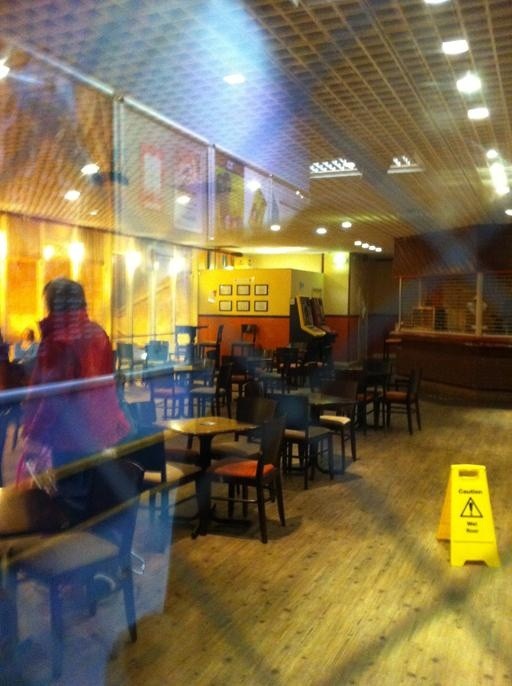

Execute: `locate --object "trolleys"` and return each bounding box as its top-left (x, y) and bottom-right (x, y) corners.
top-left (18, 450), bottom-right (144, 596)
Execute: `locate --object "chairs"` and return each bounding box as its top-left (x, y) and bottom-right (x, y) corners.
top-left (316, 378), bottom-right (360, 474)
top-left (202, 411), bottom-right (291, 543)
top-left (116, 324), bottom-right (431, 435)
top-left (165, 444), bottom-right (199, 464)
top-left (270, 392), bottom-right (335, 489)
top-left (209, 399), bottom-right (275, 517)
top-left (142, 461), bottom-right (199, 524)
top-left (2, 456), bottom-right (146, 679)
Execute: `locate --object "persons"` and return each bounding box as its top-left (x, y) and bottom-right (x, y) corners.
top-left (13, 326), bottom-right (39, 377)
top-left (1, 326), bottom-right (11, 363)
top-left (18, 274), bottom-right (132, 534)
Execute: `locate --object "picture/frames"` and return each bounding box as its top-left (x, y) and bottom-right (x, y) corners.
top-left (254, 300), bottom-right (268, 312)
top-left (236, 300), bottom-right (250, 311)
top-left (237, 285), bottom-right (250, 295)
top-left (254, 284), bottom-right (268, 295)
top-left (218, 284), bottom-right (232, 296)
top-left (218, 300), bottom-right (232, 311)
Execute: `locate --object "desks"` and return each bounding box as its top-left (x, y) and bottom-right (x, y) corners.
top-left (150, 416), bottom-right (263, 541)
